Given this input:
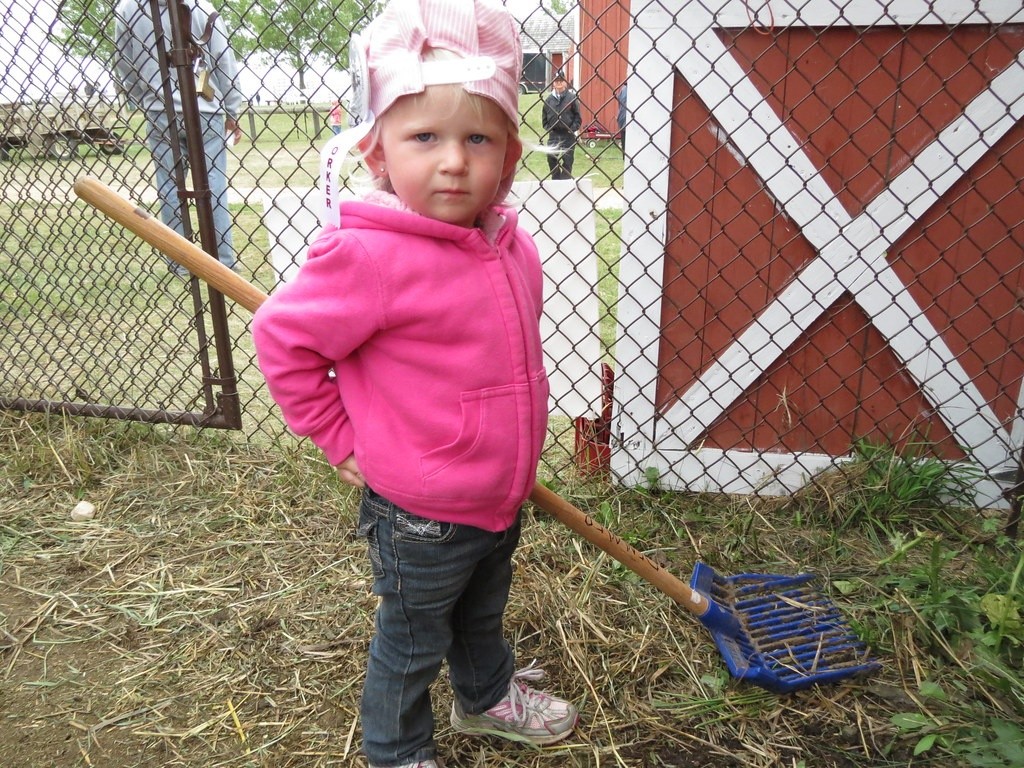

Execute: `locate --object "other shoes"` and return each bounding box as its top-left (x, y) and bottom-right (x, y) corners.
top-left (229, 264), bottom-right (240, 271)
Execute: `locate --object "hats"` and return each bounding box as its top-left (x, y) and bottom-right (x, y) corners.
top-left (349, 0), bottom-right (521, 209)
top-left (553, 70), bottom-right (565, 82)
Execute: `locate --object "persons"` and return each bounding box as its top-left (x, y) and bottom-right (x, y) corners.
top-left (617, 72), bottom-right (626, 160)
top-left (541, 71), bottom-right (582, 178)
top-left (115, 1), bottom-right (242, 273)
top-left (251, 0), bottom-right (586, 768)
top-left (331, 100), bottom-right (341, 134)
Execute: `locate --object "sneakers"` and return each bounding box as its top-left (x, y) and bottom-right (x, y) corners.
top-left (450, 680), bottom-right (579, 745)
top-left (368, 758), bottom-right (441, 768)
